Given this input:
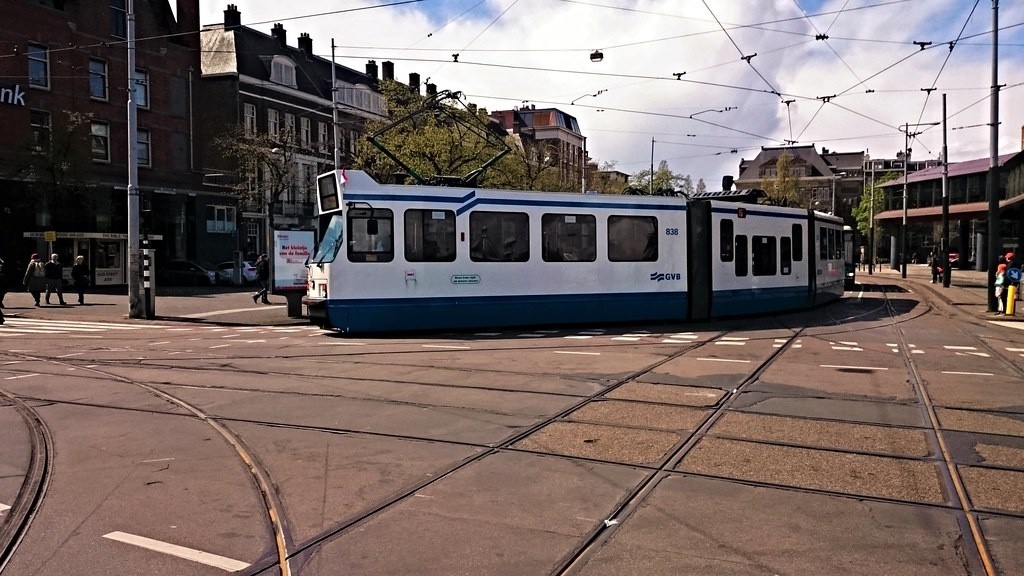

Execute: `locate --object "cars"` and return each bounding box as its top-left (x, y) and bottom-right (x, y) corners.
top-left (163, 259), bottom-right (231, 287)
top-left (949, 254), bottom-right (960, 269)
top-left (213, 261), bottom-right (260, 289)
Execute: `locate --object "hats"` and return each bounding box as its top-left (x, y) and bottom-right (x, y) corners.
top-left (1004, 253), bottom-right (1015, 259)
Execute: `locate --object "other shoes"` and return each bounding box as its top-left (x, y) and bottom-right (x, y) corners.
top-left (252, 293), bottom-right (272, 305)
top-left (995, 311), bottom-right (1015, 316)
top-left (1, 300), bottom-right (84, 325)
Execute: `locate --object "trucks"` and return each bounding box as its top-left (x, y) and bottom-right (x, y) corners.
top-left (23, 231), bottom-right (163, 293)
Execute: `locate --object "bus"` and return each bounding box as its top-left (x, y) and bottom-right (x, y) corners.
top-left (300, 169), bottom-right (857, 339)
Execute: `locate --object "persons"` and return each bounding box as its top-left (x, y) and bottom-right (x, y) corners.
top-left (250, 253), bottom-right (272, 306)
top-left (70, 256), bottom-right (90, 305)
top-left (23, 253), bottom-right (46, 306)
top-left (0, 259), bottom-right (9, 308)
top-left (994, 264), bottom-right (1007, 315)
top-left (44, 253), bottom-right (67, 305)
top-left (928, 251), bottom-right (939, 284)
top-left (997, 252), bottom-right (1017, 316)
top-left (0, 309), bottom-right (5, 325)
